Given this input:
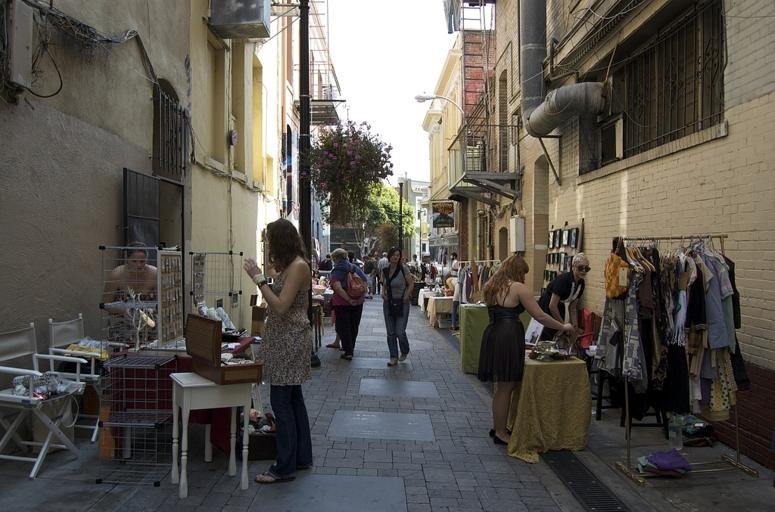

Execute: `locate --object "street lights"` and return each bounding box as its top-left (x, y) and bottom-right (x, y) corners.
top-left (397, 178), bottom-right (405, 259)
top-left (418, 209), bottom-right (426, 261)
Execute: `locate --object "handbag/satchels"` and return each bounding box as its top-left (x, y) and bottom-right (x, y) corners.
top-left (390, 299), bottom-right (403, 317)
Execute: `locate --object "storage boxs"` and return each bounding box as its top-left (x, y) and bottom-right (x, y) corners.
top-left (238, 429), bottom-right (277, 460)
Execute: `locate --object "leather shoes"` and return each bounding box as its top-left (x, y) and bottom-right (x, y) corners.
top-left (489, 429), bottom-right (509, 445)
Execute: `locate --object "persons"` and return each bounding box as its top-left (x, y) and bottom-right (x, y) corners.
top-left (536, 253), bottom-right (591, 340)
top-left (316, 247), bottom-right (465, 369)
top-left (104, 241), bottom-right (158, 359)
top-left (477, 255), bottom-right (573, 447)
top-left (242, 217), bottom-right (313, 484)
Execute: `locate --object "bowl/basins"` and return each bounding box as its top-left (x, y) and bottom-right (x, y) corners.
top-left (313, 288), bottom-right (326, 295)
top-left (537, 340), bottom-right (557, 352)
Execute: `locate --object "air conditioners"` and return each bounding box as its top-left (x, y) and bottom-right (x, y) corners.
top-left (210, 0), bottom-right (271, 39)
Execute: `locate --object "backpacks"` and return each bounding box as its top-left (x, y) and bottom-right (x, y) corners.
top-left (332, 264), bottom-right (365, 299)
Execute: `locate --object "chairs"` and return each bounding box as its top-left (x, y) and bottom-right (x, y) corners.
top-left (47, 314), bottom-right (130, 442)
top-left (0, 323), bottom-right (86, 479)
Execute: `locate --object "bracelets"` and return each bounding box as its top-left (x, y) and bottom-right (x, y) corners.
top-left (253, 273), bottom-right (267, 287)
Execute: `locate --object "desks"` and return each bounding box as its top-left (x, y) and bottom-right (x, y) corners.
top-left (108, 338), bottom-right (256, 462)
top-left (459, 302), bottom-right (532, 374)
top-left (509, 344), bottom-right (597, 466)
top-left (167, 373), bottom-right (254, 498)
top-left (418, 288), bottom-right (456, 328)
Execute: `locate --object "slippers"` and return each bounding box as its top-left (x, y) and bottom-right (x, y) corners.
top-left (256, 474), bottom-right (295, 484)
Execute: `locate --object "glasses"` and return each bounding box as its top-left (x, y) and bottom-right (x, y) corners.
top-left (578, 266), bottom-right (590, 271)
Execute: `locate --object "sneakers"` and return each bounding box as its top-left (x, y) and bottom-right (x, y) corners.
top-left (387, 353), bottom-right (407, 365)
top-left (327, 344), bottom-right (353, 359)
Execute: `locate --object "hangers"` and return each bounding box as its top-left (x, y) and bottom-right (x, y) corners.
top-left (608, 235), bottom-right (719, 272)
top-left (459, 260), bottom-right (500, 271)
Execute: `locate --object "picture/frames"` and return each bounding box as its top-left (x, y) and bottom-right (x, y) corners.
top-left (541, 228), bottom-right (578, 295)
